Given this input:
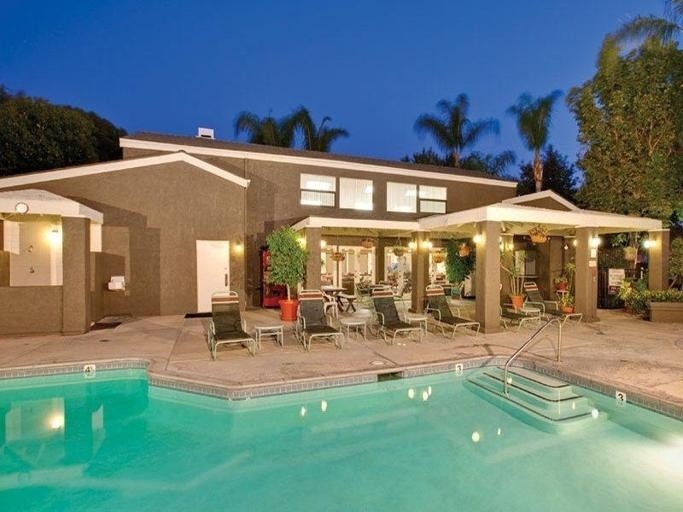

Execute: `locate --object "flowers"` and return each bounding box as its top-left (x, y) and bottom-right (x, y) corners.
top-left (328, 238), bottom-right (470, 263)
top-left (527, 222), bottom-right (551, 239)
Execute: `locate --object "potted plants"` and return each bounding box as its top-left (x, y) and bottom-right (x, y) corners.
top-left (556, 285), bottom-right (568, 311)
top-left (549, 261), bottom-right (578, 293)
top-left (262, 223), bottom-right (310, 326)
top-left (506, 249), bottom-right (529, 310)
top-left (563, 296), bottom-right (574, 314)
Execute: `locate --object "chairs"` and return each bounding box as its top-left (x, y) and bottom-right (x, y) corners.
top-left (202, 290), bottom-right (259, 362)
top-left (421, 280), bottom-right (481, 347)
top-left (491, 284), bottom-right (536, 332)
top-left (522, 278), bottom-right (566, 321)
top-left (292, 289), bottom-right (344, 354)
top-left (364, 287), bottom-right (425, 345)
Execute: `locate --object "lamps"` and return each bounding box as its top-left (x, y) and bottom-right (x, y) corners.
top-left (38, 220), bottom-right (66, 250)
top-left (233, 240), bottom-right (246, 257)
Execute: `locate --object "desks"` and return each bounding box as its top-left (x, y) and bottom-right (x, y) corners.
top-left (396, 307), bottom-right (429, 337)
top-left (250, 318), bottom-right (288, 351)
top-left (338, 317), bottom-right (371, 344)
